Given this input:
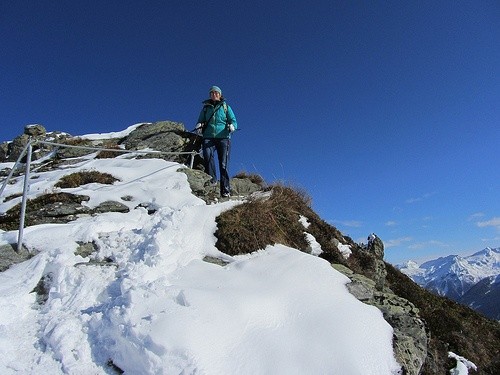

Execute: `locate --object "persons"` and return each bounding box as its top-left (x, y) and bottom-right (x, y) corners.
top-left (194, 85), bottom-right (237, 201)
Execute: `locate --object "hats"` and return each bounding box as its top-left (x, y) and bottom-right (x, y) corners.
top-left (208, 85), bottom-right (222, 97)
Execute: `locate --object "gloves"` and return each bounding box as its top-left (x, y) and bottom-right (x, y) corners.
top-left (227, 124), bottom-right (235, 131)
top-left (195, 123), bottom-right (203, 133)
